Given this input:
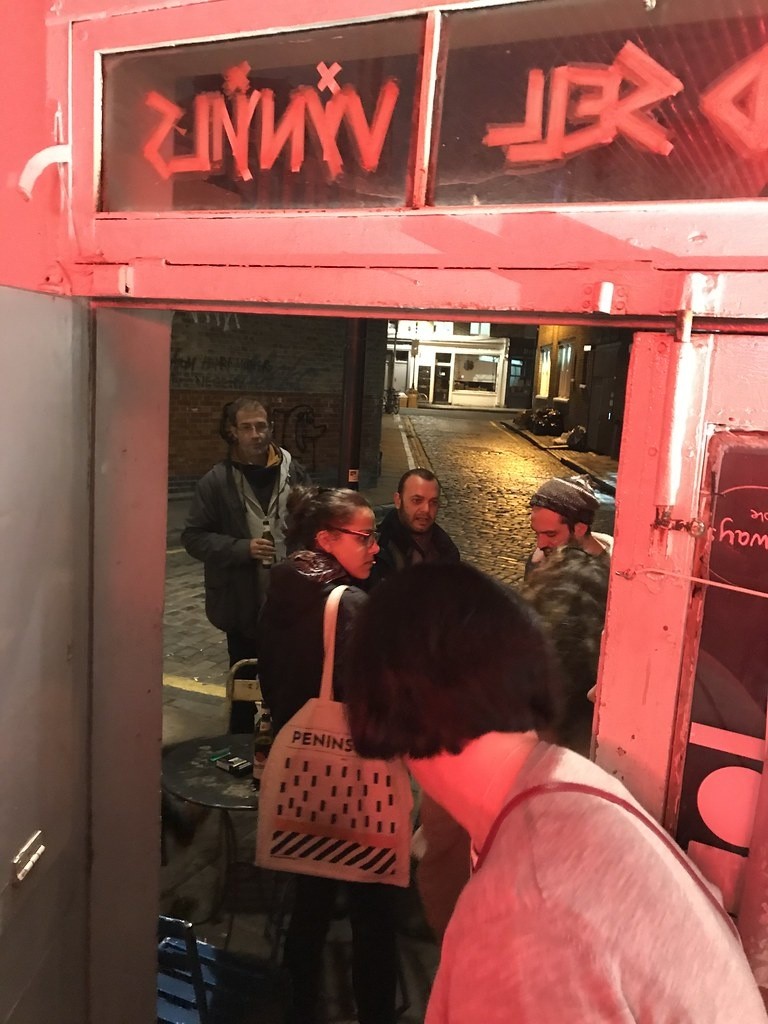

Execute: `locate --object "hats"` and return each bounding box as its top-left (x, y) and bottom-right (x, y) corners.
top-left (530, 474), bottom-right (600, 526)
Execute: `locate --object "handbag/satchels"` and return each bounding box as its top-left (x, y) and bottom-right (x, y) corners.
top-left (256, 585), bottom-right (410, 887)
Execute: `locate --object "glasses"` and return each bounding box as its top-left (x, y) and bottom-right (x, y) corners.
top-left (235, 422), bottom-right (271, 434)
top-left (323, 524), bottom-right (381, 548)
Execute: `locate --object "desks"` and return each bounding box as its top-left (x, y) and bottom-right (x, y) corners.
top-left (160, 732), bottom-right (278, 877)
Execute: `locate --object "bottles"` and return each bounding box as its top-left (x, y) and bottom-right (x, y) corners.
top-left (253, 700), bottom-right (274, 785)
top-left (261, 520), bottom-right (276, 568)
top-left (347, 450), bottom-right (359, 490)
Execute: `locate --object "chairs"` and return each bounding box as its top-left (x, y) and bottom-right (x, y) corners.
top-left (225, 658), bottom-right (271, 732)
top-left (156, 913), bottom-right (281, 1024)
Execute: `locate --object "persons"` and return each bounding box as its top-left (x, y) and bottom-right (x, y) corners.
top-left (525, 474), bottom-right (614, 584)
top-left (181, 397), bottom-right (316, 732)
top-left (254, 483), bottom-right (397, 1024)
top-left (414, 564), bottom-right (611, 949)
top-left (342, 563), bottom-right (768, 1024)
top-left (358, 468), bottom-right (460, 590)
